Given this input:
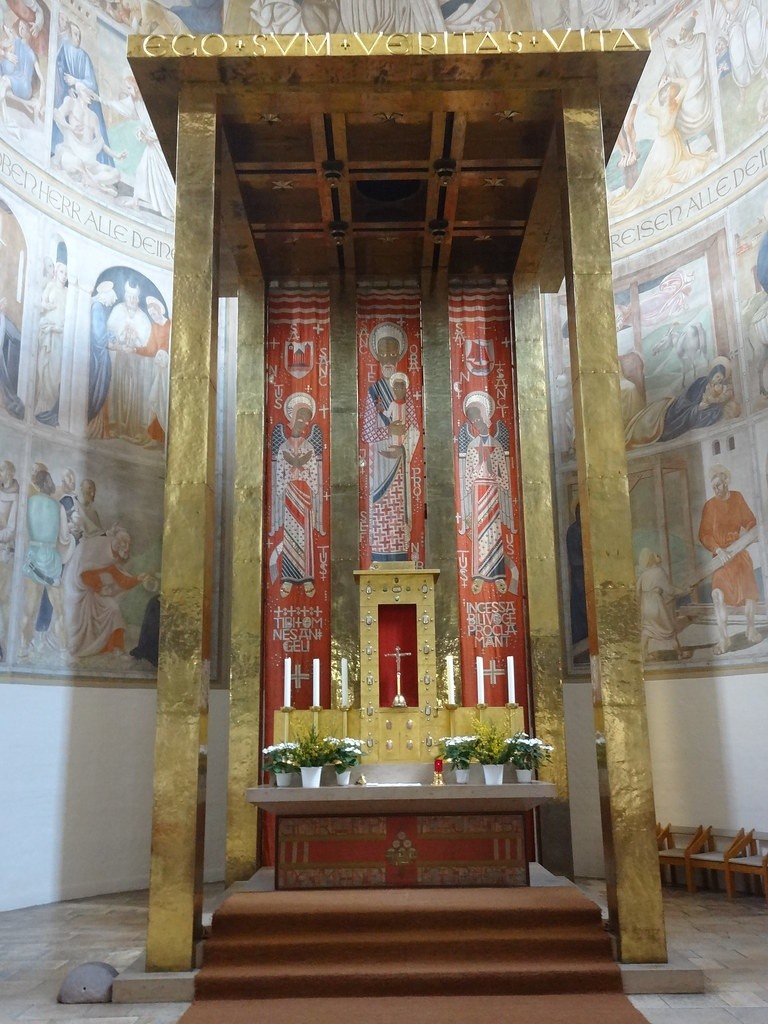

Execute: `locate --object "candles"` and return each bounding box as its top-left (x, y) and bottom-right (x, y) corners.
top-left (476, 656), bottom-right (485, 704)
top-left (312, 658), bottom-right (320, 707)
top-left (341, 657), bottom-right (348, 706)
top-left (446, 655), bottom-right (456, 704)
top-left (284, 656), bottom-right (291, 707)
top-left (507, 654), bottom-right (515, 703)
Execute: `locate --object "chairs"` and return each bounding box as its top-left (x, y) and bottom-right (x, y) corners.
top-left (656, 822), bottom-right (709, 893)
top-left (725, 827), bottom-right (768, 904)
top-left (686, 824), bottom-right (752, 899)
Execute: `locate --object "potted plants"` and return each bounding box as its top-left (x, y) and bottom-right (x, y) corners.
top-left (282, 723), bottom-right (339, 788)
top-left (470, 712), bottom-right (524, 784)
top-left (435, 736), bottom-right (479, 783)
top-left (322, 735), bottom-right (368, 786)
top-left (505, 731), bottom-right (554, 783)
top-left (261, 740), bottom-right (300, 787)
